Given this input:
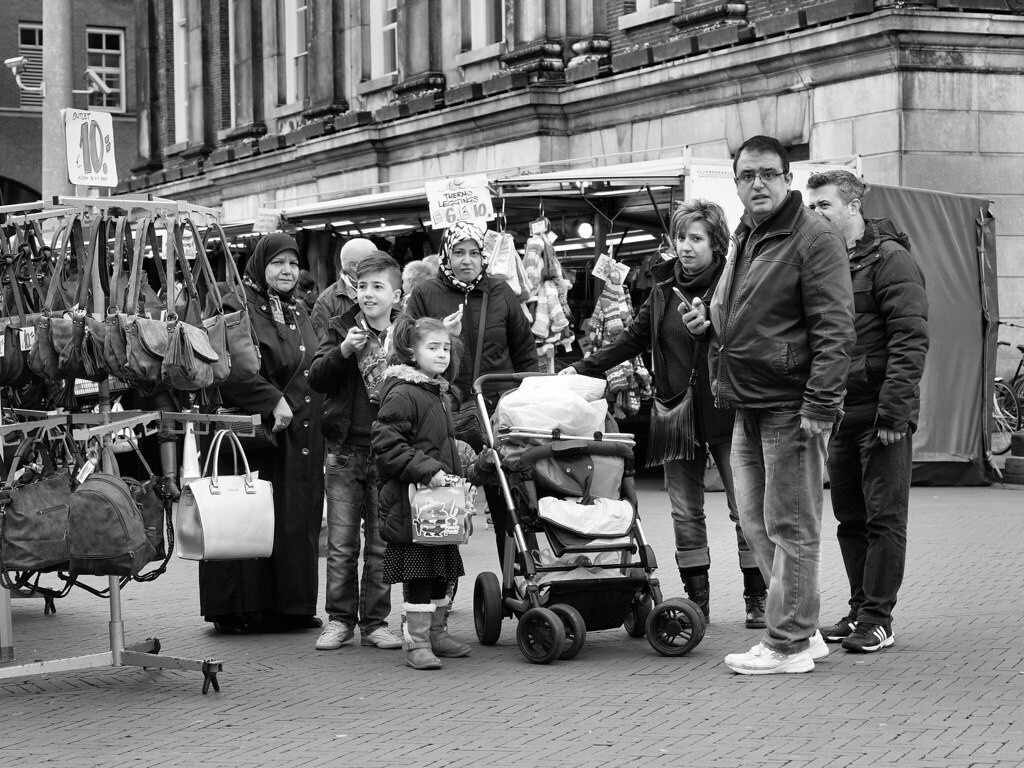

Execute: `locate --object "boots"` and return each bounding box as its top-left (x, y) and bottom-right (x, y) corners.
top-left (430, 595), bottom-right (472, 657)
top-left (402, 603), bottom-right (443, 669)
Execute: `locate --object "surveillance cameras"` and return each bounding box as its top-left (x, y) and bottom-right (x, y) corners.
top-left (84, 69), bottom-right (112, 95)
top-left (4, 56), bottom-right (29, 69)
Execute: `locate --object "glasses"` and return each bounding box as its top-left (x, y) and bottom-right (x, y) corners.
top-left (735, 171), bottom-right (785, 185)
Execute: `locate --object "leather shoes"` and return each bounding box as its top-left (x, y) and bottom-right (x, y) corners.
top-left (667, 588), bottom-right (711, 626)
top-left (743, 592), bottom-right (768, 628)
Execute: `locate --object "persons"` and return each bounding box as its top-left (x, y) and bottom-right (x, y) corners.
top-left (677, 134), bottom-right (857, 674)
top-left (203, 221), bottom-right (540, 669)
top-left (558, 198), bottom-right (771, 629)
top-left (805, 168), bottom-right (929, 652)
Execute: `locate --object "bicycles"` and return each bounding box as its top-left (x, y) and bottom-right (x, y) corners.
top-left (988, 340), bottom-right (1024, 455)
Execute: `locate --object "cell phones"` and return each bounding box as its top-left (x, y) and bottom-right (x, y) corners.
top-left (671, 285), bottom-right (704, 327)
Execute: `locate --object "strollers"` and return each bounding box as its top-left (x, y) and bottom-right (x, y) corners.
top-left (472, 371), bottom-right (707, 665)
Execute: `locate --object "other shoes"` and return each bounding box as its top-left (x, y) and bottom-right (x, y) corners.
top-left (213, 618), bottom-right (250, 635)
top-left (274, 613), bottom-right (323, 627)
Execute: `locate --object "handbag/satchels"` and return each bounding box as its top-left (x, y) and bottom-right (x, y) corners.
top-left (407, 474), bottom-right (476, 545)
top-left (0, 213), bottom-right (274, 618)
top-left (448, 398), bottom-right (488, 457)
top-left (645, 385), bottom-right (700, 467)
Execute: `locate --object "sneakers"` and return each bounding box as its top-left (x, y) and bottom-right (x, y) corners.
top-left (842, 621), bottom-right (895, 651)
top-left (315, 620), bottom-right (355, 649)
top-left (724, 642), bottom-right (815, 674)
top-left (360, 627), bottom-right (403, 648)
top-left (808, 626), bottom-right (830, 660)
top-left (817, 615), bottom-right (859, 642)
